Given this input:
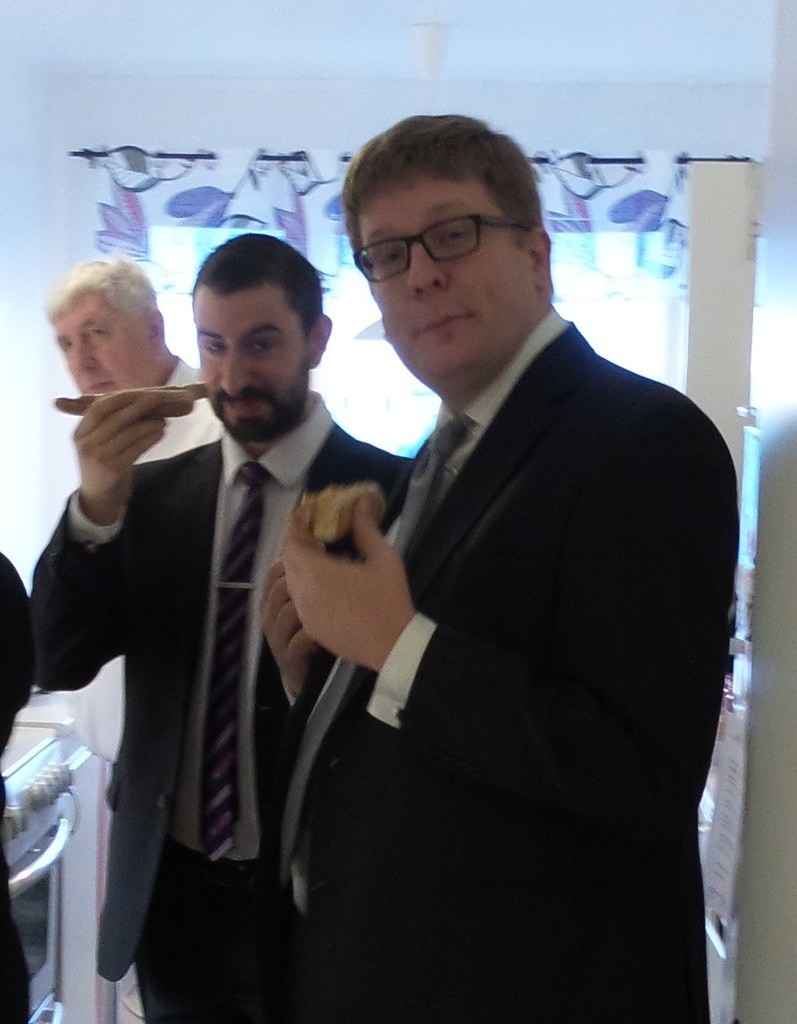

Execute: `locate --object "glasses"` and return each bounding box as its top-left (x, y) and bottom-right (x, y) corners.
top-left (352, 212), bottom-right (531, 285)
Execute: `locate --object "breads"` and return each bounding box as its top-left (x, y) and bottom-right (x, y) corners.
top-left (56, 382), bottom-right (206, 419)
top-left (300, 482), bottom-right (385, 542)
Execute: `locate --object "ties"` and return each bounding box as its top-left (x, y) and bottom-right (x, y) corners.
top-left (200, 461), bottom-right (268, 860)
top-left (277, 416), bottom-right (476, 887)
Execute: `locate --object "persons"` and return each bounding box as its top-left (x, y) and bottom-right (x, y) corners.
top-left (33, 235), bottom-right (410, 1024)
top-left (0, 552), bottom-right (35, 1024)
top-left (261, 116), bottom-right (740, 1024)
top-left (44, 261), bottom-right (223, 764)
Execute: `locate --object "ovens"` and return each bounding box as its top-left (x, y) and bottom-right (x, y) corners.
top-left (0, 734), bottom-right (90, 1024)
